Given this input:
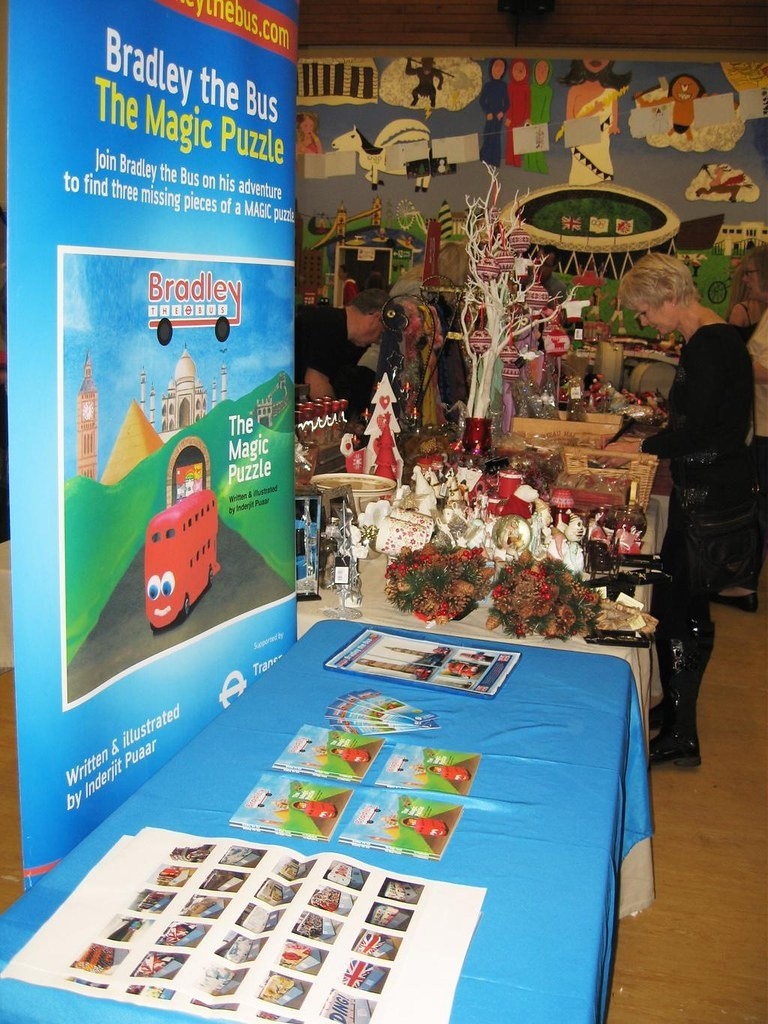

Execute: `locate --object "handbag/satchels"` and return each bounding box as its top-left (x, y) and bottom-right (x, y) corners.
top-left (684, 497), bottom-right (764, 603)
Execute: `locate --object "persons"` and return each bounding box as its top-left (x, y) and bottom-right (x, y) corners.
top-left (334, 241), bottom-right (470, 424)
top-left (710, 242), bottom-right (767, 613)
top-left (529, 242), bottom-right (570, 308)
top-left (295, 287), bottom-right (396, 401)
top-left (596, 254), bottom-right (755, 768)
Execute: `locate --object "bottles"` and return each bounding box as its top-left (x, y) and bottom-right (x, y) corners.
top-left (416, 458), bottom-right (432, 482)
top-left (295, 396), bottom-right (347, 446)
top-left (325, 518), bottom-right (341, 538)
top-left (431, 455), bottom-right (443, 474)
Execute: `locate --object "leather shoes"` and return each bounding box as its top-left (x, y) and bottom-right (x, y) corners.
top-left (709, 585), bottom-right (758, 613)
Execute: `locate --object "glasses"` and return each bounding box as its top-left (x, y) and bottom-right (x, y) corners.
top-left (741, 269), bottom-right (759, 274)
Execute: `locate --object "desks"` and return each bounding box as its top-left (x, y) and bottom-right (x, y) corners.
top-left (295, 497), bottom-right (660, 757)
top-left (0, 621), bottom-right (658, 1024)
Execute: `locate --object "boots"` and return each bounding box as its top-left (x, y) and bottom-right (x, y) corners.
top-left (649, 617), bottom-right (715, 766)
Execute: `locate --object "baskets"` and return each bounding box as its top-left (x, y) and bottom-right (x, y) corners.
top-left (564, 447), bottom-right (659, 516)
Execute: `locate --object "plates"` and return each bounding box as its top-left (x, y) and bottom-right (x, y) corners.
top-left (310, 473), bottom-right (395, 492)
